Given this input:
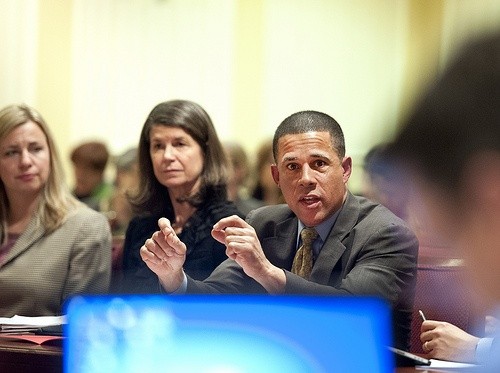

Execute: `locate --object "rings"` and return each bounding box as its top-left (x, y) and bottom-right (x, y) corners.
top-left (424, 340), bottom-right (430, 352)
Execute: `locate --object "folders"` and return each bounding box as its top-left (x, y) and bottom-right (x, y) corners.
top-left (390, 345), bottom-right (433, 366)
top-left (0, 314), bottom-right (63, 335)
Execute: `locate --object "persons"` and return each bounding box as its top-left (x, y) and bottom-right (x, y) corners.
top-left (420, 306), bottom-right (500, 373)
top-left (68, 138), bottom-right (283, 233)
top-left (109, 99), bottom-right (246, 296)
top-left (0, 103), bottom-right (112, 318)
top-left (139, 109), bottom-right (420, 367)
top-left (352, 142), bottom-right (427, 247)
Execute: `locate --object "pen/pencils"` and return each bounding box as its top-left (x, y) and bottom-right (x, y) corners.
top-left (417, 308), bottom-right (431, 324)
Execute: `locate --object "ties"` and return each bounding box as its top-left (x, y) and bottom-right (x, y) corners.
top-left (291, 229), bottom-right (319, 279)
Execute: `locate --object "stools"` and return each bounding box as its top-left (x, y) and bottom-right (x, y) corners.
top-left (411, 258), bottom-right (488, 354)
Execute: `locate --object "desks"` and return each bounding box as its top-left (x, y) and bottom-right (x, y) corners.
top-left (0, 345), bottom-right (500, 373)
